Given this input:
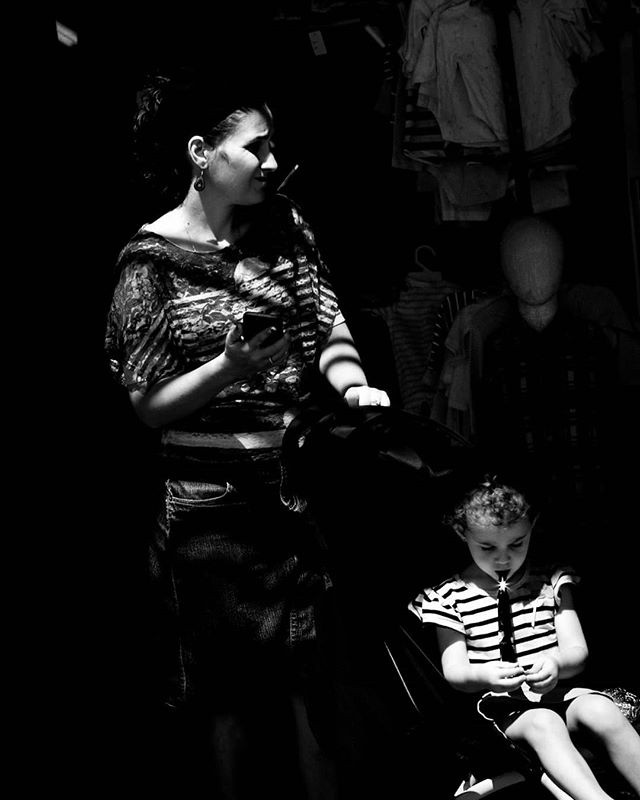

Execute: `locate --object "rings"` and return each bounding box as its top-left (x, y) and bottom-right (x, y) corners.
top-left (268, 355), bottom-right (275, 365)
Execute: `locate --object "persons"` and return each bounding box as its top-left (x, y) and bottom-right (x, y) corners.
top-left (103, 71), bottom-right (392, 800)
top-left (464, 216), bottom-right (640, 690)
top-left (407, 474), bottom-right (639, 800)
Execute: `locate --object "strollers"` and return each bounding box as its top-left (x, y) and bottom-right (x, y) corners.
top-left (281, 396), bottom-right (640, 800)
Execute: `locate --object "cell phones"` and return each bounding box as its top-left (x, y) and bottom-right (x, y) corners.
top-left (242, 311), bottom-right (284, 348)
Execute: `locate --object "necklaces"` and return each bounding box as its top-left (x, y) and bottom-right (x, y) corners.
top-left (185, 223), bottom-right (196, 252)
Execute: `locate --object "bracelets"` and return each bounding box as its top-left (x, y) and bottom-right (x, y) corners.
top-left (339, 383), bottom-right (362, 396)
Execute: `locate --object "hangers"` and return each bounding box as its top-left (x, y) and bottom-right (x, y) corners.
top-left (403, 244), bottom-right (459, 289)
